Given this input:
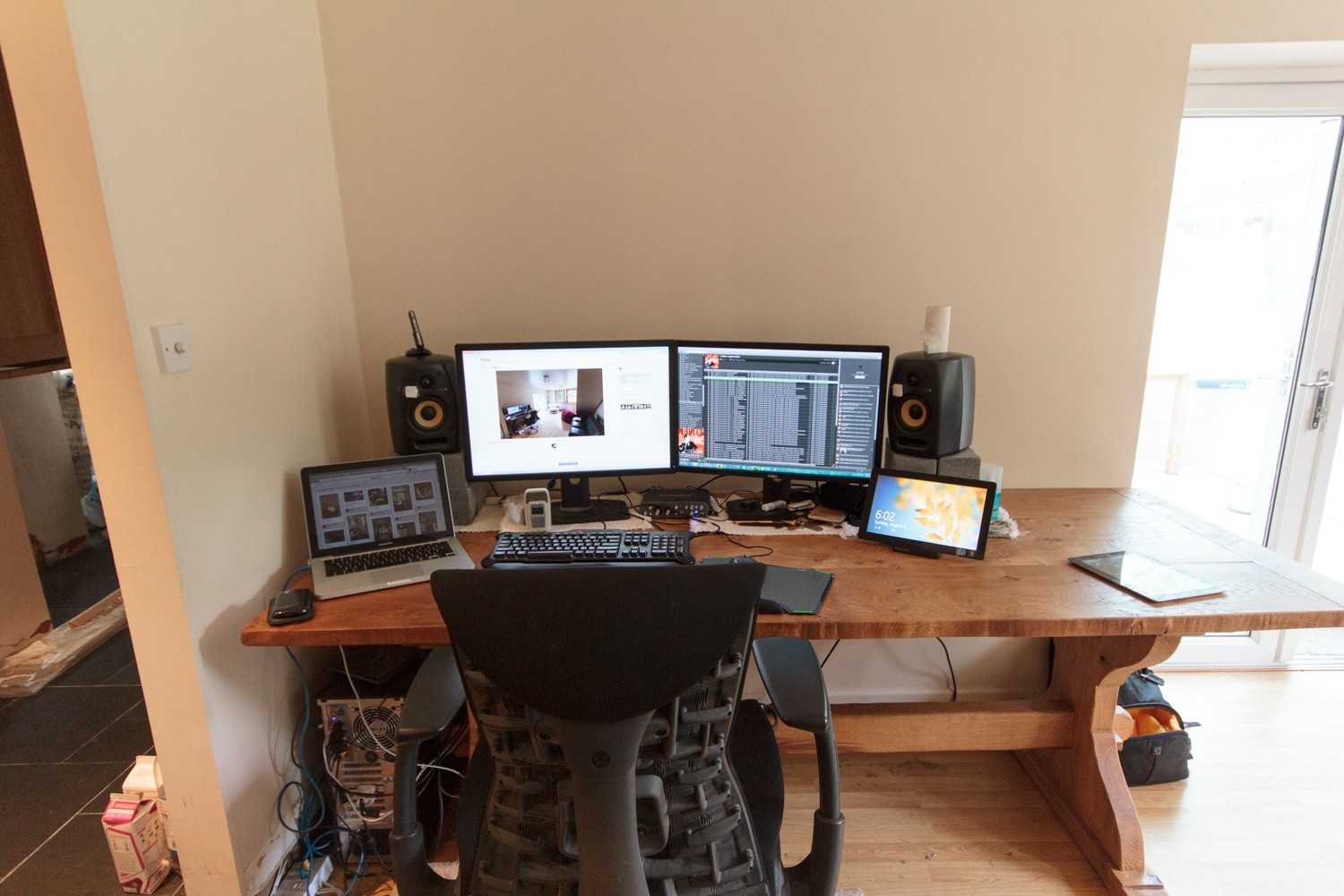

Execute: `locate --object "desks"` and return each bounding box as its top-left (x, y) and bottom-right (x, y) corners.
top-left (239, 482), bottom-right (1344, 896)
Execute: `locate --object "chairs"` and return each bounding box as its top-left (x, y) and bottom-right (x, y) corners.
top-left (388, 562), bottom-right (846, 895)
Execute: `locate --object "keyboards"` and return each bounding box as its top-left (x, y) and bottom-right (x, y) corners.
top-left (480, 529), bottom-right (696, 568)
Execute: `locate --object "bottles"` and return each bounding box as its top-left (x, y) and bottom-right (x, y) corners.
top-left (154, 754), bottom-right (182, 880)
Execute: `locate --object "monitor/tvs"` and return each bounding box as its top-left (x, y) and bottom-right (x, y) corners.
top-left (453, 339), bottom-right (680, 526)
top-left (675, 339), bottom-right (890, 526)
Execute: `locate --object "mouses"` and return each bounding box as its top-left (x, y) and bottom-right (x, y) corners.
top-left (729, 556), bottom-right (756, 564)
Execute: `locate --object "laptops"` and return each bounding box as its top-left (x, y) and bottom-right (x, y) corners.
top-left (299, 452), bottom-right (476, 601)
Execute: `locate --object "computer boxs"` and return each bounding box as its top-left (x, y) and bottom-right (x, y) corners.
top-left (316, 646), bottom-right (442, 865)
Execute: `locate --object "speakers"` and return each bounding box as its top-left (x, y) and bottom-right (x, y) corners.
top-left (384, 353), bottom-right (464, 456)
top-left (887, 351), bottom-right (975, 459)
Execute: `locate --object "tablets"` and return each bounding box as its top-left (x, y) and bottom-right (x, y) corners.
top-left (1068, 548), bottom-right (1227, 603)
top-left (856, 467), bottom-right (998, 561)
